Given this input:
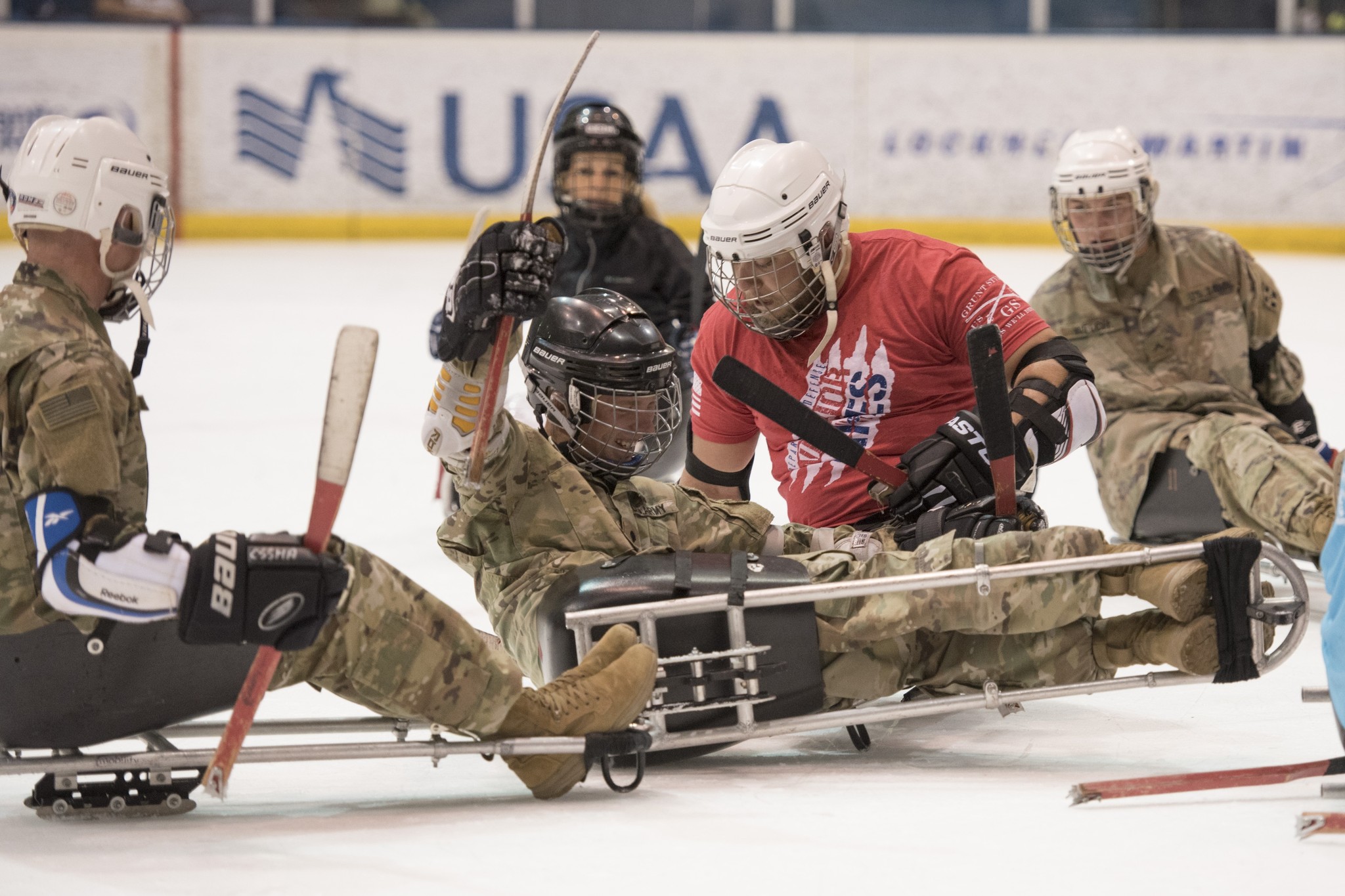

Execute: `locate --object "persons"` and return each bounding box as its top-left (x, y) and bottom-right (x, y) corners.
top-left (1029, 127), bottom-right (1345, 573)
top-left (0, 114), bottom-right (655, 801)
top-left (420, 219), bottom-right (1281, 766)
top-left (677, 134), bottom-right (1101, 554)
top-left (530, 96), bottom-right (716, 483)
top-left (1320, 462), bottom-right (1345, 750)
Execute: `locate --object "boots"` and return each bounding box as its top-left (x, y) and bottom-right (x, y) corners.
top-left (483, 643), bottom-right (657, 801)
top-left (523, 623), bottom-right (636, 695)
top-left (1092, 581), bottom-right (1275, 675)
top-left (1099, 527), bottom-right (1257, 623)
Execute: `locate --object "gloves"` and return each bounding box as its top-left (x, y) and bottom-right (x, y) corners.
top-left (867, 405), bottom-right (1034, 523)
top-left (176, 530), bottom-right (355, 651)
top-left (893, 494), bottom-right (1048, 552)
top-left (429, 220), bottom-right (560, 362)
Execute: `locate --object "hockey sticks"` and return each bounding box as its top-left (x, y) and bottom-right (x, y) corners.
top-left (1061, 754), bottom-right (1345, 807)
top-left (462, 30), bottom-right (603, 484)
top-left (709, 356), bottom-right (909, 491)
top-left (199, 324), bottom-right (381, 799)
top-left (966, 321), bottom-right (1020, 519)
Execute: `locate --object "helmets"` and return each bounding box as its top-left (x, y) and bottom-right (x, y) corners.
top-left (1047, 126), bottom-right (1159, 274)
top-left (6, 115), bottom-right (175, 324)
top-left (700, 138), bottom-right (852, 343)
top-left (521, 288), bottom-right (683, 486)
top-left (551, 100), bottom-right (645, 228)
